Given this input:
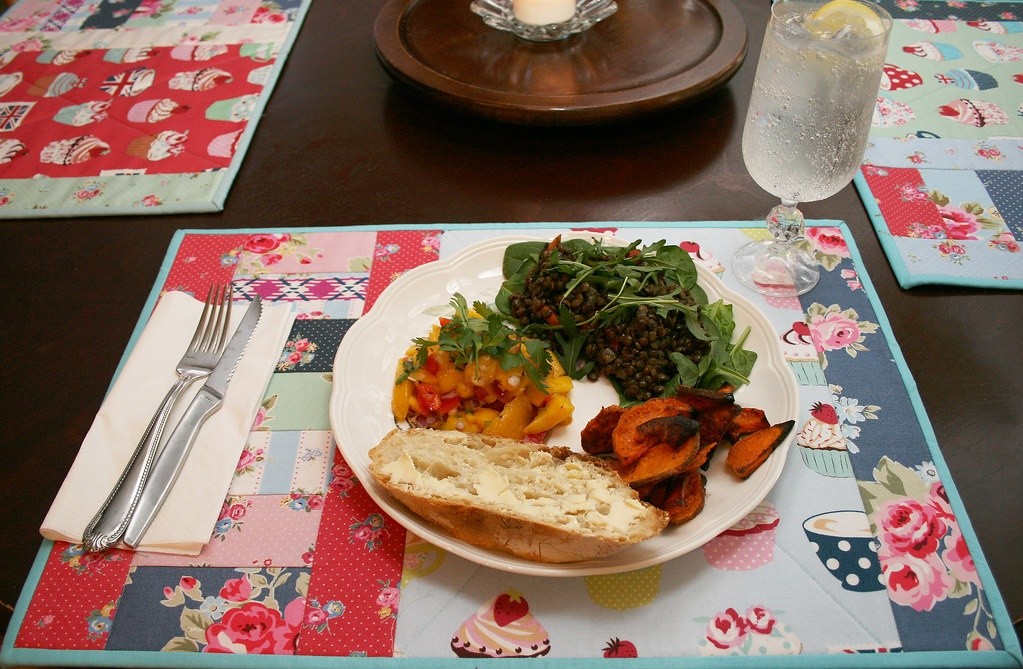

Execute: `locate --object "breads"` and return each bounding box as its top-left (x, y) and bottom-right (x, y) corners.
top-left (367, 427), bottom-right (670, 562)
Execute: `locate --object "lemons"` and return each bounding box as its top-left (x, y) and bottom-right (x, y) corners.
top-left (807, 0), bottom-right (884, 46)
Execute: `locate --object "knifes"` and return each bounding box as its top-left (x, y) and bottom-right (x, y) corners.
top-left (124, 294), bottom-right (263, 549)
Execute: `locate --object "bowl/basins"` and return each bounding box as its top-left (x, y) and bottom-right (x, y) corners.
top-left (470, 0), bottom-right (621, 42)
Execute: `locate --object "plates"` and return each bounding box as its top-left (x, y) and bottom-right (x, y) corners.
top-left (330, 235), bottom-right (800, 576)
top-left (373, 1), bottom-right (750, 122)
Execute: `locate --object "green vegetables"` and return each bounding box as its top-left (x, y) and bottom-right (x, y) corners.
top-left (392, 238), bottom-right (756, 406)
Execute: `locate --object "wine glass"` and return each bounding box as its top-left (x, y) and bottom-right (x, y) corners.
top-left (731, 1), bottom-right (895, 297)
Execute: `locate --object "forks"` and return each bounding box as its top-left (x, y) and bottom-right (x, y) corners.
top-left (81, 283), bottom-right (232, 549)
